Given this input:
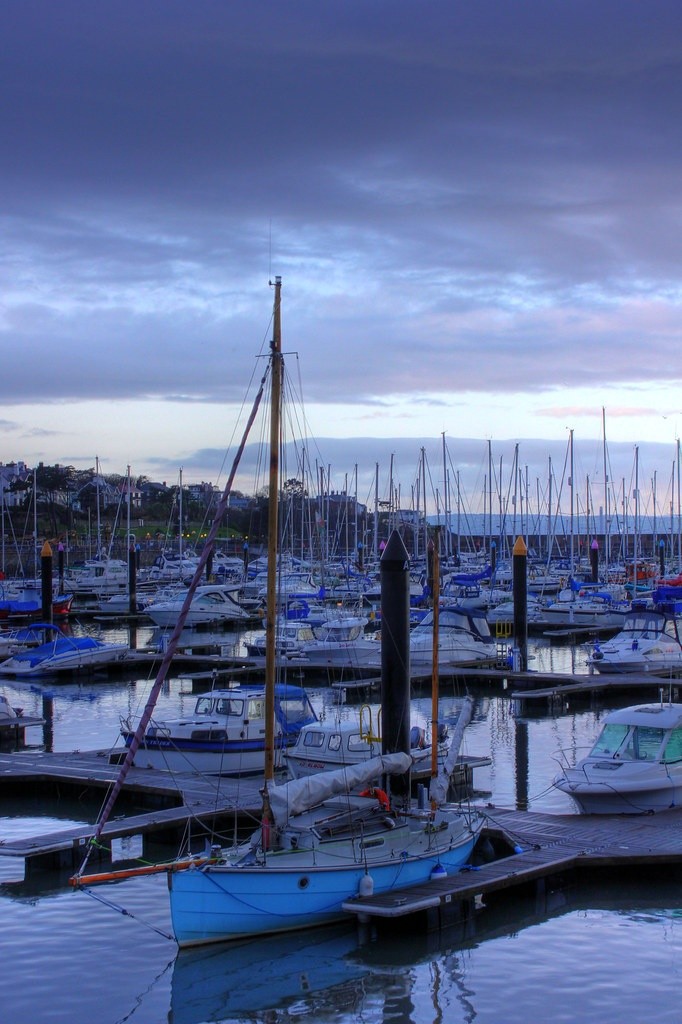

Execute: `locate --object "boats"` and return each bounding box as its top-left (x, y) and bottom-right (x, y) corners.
top-left (552, 689), bottom-right (676, 813)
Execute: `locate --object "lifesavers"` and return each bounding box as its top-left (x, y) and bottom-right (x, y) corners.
top-left (559, 578), bottom-right (567, 588)
top-left (530, 575), bottom-right (535, 580)
top-left (360, 788), bottom-right (390, 811)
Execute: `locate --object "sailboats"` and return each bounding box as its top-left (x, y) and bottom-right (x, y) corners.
top-left (68, 277), bottom-right (492, 944)
top-left (2, 407), bottom-right (680, 776)
